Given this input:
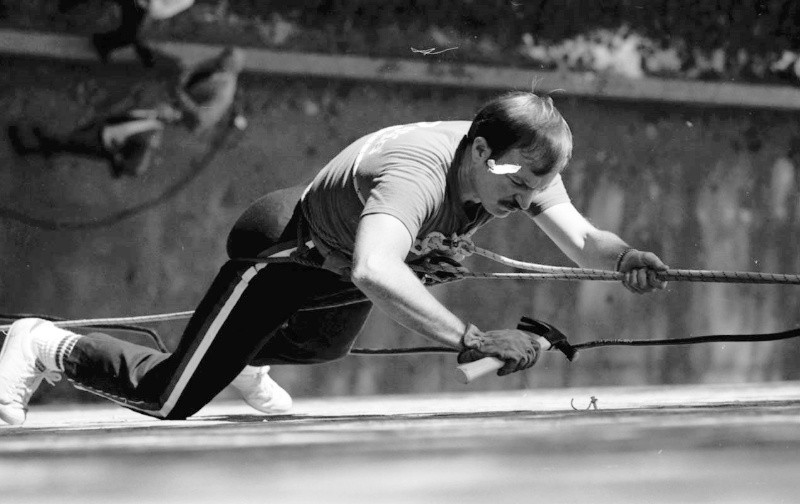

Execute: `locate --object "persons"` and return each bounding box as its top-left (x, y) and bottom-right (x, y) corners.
top-left (114, 45), bottom-right (243, 134)
top-left (89, 0), bottom-right (193, 61)
top-left (8, 103), bottom-right (187, 177)
top-left (0, 93), bottom-right (670, 426)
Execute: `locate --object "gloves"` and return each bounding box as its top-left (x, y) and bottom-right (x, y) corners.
top-left (457, 322), bottom-right (541, 376)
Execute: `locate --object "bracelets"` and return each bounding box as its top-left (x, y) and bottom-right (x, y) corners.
top-left (615, 249), bottom-right (637, 272)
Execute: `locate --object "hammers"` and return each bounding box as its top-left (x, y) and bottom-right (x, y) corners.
top-left (455, 316), bottom-right (577, 384)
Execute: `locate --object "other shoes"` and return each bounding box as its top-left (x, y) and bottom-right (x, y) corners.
top-left (93, 33), bottom-right (110, 66)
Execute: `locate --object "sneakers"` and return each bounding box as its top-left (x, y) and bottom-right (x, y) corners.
top-left (0, 318), bottom-right (63, 425)
top-left (231, 364), bottom-right (292, 413)
